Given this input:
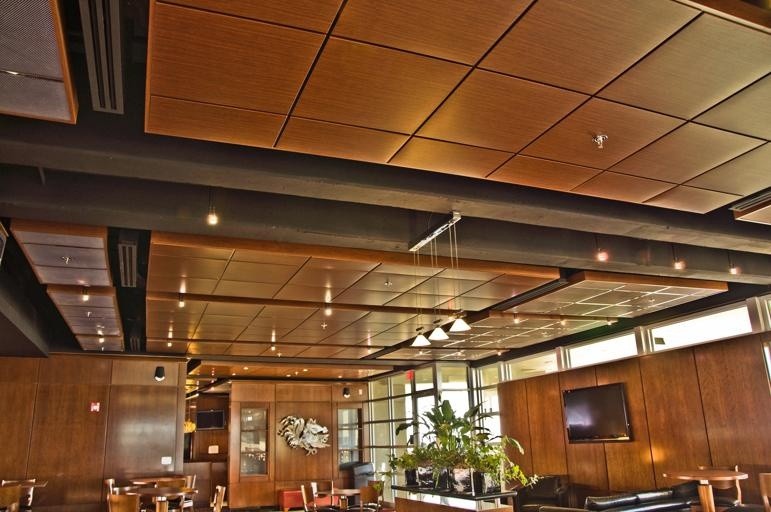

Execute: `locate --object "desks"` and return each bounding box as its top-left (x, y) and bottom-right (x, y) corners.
top-left (662, 467), bottom-right (748, 512)
top-left (3, 480), bottom-right (49, 488)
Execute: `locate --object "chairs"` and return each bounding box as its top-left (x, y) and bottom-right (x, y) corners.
top-left (300, 479), bottom-right (384, 512)
top-left (102, 474), bottom-right (227, 512)
top-left (514, 475), bottom-right (569, 511)
top-left (0, 479), bottom-right (36, 512)
top-left (690, 464), bottom-right (771, 512)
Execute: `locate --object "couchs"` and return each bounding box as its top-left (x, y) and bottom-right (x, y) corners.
top-left (539, 480), bottom-right (733, 512)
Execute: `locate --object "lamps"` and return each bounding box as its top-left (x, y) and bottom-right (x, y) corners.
top-left (407, 208), bottom-right (472, 348)
top-left (154, 366), bottom-right (165, 382)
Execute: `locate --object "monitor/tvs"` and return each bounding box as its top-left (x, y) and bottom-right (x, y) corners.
top-left (196, 410), bottom-right (224, 429)
top-left (563, 383), bottom-right (632, 443)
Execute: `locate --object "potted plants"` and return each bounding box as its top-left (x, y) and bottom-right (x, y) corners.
top-left (388, 394), bottom-right (546, 496)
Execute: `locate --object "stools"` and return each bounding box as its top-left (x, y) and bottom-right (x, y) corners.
top-left (278, 488), bottom-right (312, 512)
